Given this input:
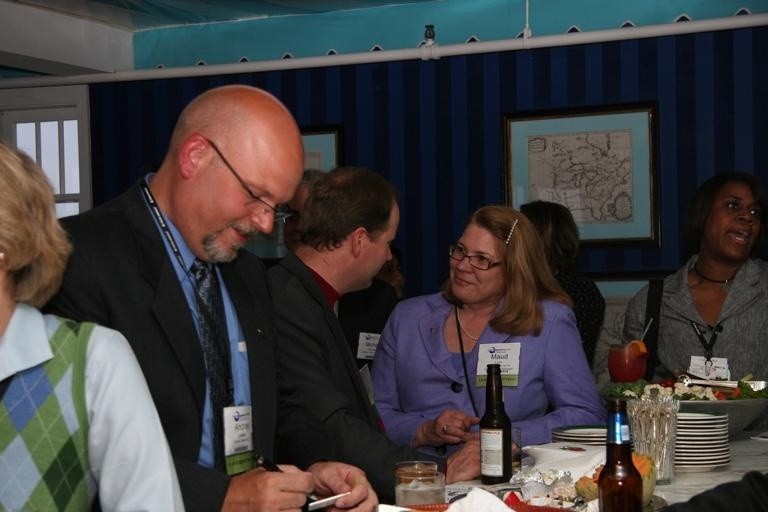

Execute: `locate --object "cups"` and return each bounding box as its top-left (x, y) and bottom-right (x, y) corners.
top-left (394, 460), bottom-right (437, 484)
top-left (395, 471), bottom-right (446, 510)
top-left (628, 400), bottom-right (677, 487)
top-left (510, 428), bottom-right (521, 474)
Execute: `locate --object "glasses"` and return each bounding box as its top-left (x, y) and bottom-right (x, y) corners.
top-left (207, 139), bottom-right (294, 222)
top-left (448, 241), bottom-right (502, 271)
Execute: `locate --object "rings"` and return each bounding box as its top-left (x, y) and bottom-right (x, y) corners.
top-left (442, 425), bottom-right (447, 433)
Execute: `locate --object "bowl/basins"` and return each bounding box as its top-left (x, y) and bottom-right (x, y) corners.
top-left (521, 440), bottom-right (606, 468)
top-left (600, 380), bottom-right (767, 441)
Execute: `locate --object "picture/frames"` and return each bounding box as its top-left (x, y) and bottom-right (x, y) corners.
top-left (502, 104), bottom-right (661, 245)
top-left (302, 121), bottom-right (349, 174)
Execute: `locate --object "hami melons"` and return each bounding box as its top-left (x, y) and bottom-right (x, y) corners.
top-left (575, 452), bottom-right (656, 507)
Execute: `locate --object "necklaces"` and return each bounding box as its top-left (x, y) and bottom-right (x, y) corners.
top-left (693, 264), bottom-right (737, 284)
top-left (456, 306), bottom-right (480, 341)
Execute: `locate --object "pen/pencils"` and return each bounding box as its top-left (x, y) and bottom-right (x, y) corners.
top-left (250, 451), bottom-right (316, 505)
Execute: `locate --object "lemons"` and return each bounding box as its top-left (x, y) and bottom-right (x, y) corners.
top-left (625, 340), bottom-right (648, 353)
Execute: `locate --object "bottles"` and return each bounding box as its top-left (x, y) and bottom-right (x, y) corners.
top-left (598, 399), bottom-right (643, 512)
top-left (479, 364), bottom-right (512, 484)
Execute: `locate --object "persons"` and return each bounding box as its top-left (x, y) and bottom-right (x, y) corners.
top-left (269, 165), bottom-right (517, 487)
top-left (283, 167), bottom-right (399, 375)
top-left (38, 84), bottom-right (380, 512)
top-left (518, 199), bottom-right (607, 372)
top-left (593, 173), bottom-right (768, 395)
top-left (0, 141), bottom-right (186, 512)
top-left (370, 204), bottom-right (605, 458)
top-left (378, 250), bottom-right (406, 301)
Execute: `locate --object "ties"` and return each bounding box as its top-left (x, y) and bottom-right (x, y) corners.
top-left (192, 260), bottom-right (234, 474)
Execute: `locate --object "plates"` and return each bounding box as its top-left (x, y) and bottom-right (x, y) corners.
top-left (674, 412), bottom-right (731, 474)
top-left (550, 422), bottom-right (610, 446)
